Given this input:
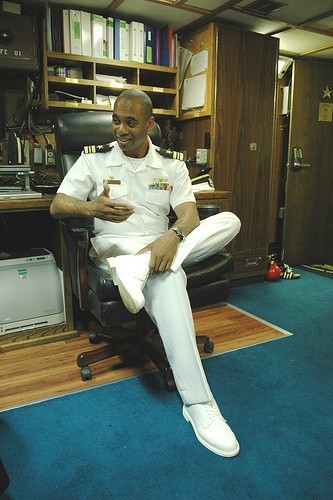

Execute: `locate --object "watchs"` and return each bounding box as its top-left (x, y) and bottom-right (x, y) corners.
top-left (169, 227), bottom-right (184, 243)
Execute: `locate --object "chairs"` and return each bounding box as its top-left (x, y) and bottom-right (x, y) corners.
top-left (52, 112), bottom-right (235, 394)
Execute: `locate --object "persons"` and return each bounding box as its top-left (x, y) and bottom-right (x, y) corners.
top-left (51, 88), bottom-right (242, 458)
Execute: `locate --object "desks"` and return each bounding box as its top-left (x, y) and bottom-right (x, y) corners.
top-left (0, 188), bottom-right (231, 331)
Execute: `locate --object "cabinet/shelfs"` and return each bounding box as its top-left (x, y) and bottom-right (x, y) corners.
top-left (35, 17), bottom-right (179, 119)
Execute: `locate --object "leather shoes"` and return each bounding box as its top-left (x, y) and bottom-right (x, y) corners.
top-left (182, 398), bottom-right (240, 458)
top-left (104, 254), bottom-right (150, 314)
